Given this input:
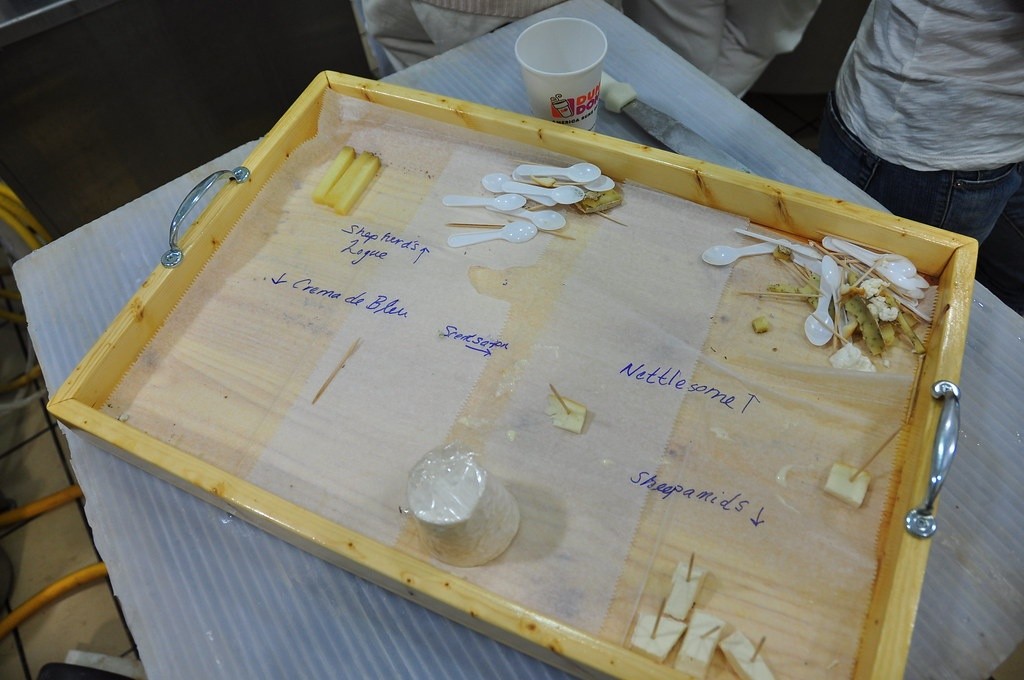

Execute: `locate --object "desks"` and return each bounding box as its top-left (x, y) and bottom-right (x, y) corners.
top-left (13, 0), bottom-right (1024, 680)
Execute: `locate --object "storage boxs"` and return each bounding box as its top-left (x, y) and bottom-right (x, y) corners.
top-left (45, 72), bottom-right (978, 680)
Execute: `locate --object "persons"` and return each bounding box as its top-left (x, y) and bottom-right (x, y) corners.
top-left (363, 0), bottom-right (574, 68)
top-left (820, 0), bottom-right (1024, 246)
top-left (623, 0), bottom-right (819, 99)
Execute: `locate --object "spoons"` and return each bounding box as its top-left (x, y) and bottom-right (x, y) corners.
top-left (485, 198), bottom-right (566, 230)
top-left (517, 162), bottom-right (601, 182)
top-left (482, 173), bottom-right (557, 206)
top-left (502, 182), bottom-right (585, 204)
top-left (513, 167), bottom-right (616, 191)
top-left (447, 221), bottom-right (538, 248)
top-left (703, 228), bottom-right (929, 347)
top-left (442, 194), bottom-right (526, 211)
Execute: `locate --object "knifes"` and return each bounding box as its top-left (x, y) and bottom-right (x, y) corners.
top-left (600, 70), bottom-right (759, 178)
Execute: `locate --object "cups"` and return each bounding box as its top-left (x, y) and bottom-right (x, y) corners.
top-left (515, 17), bottom-right (607, 135)
top-left (407, 449), bottom-right (521, 567)
